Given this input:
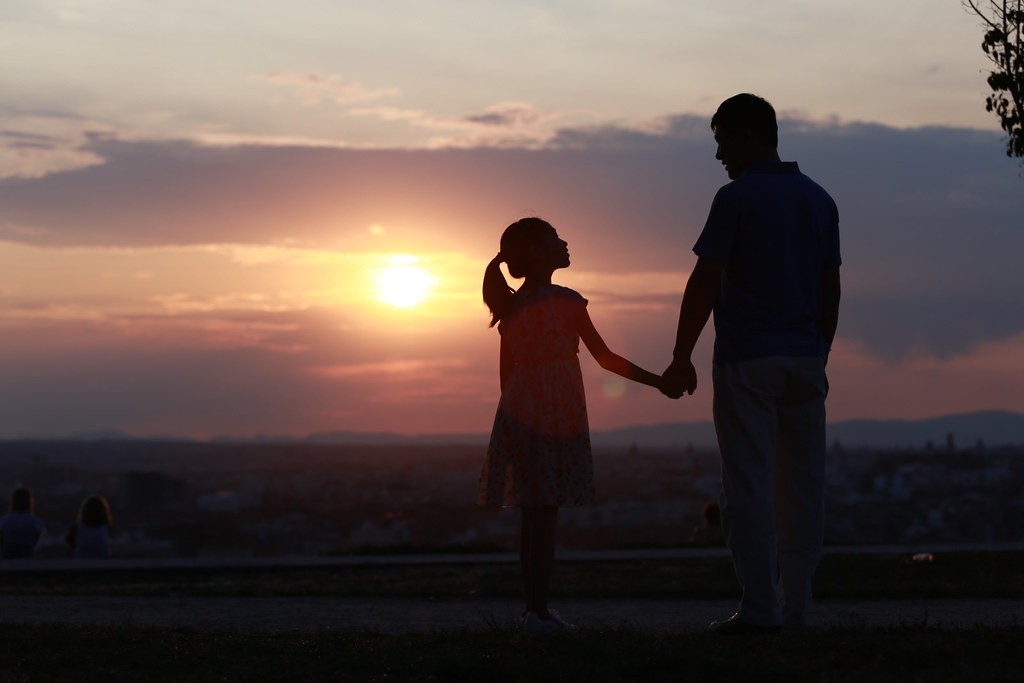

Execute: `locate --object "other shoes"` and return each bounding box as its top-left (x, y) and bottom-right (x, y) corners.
top-left (518, 612), bottom-right (576, 633)
top-left (710, 611), bottom-right (802, 636)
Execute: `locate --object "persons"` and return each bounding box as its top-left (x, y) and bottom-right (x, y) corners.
top-left (688, 496), bottom-right (724, 544)
top-left (663, 93), bottom-right (840, 632)
top-left (61, 496), bottom-right (113, 556)
top-left (0, 488), bottom-right (44, 558)
top-left (477, 216), bottom-right (686, 626)
